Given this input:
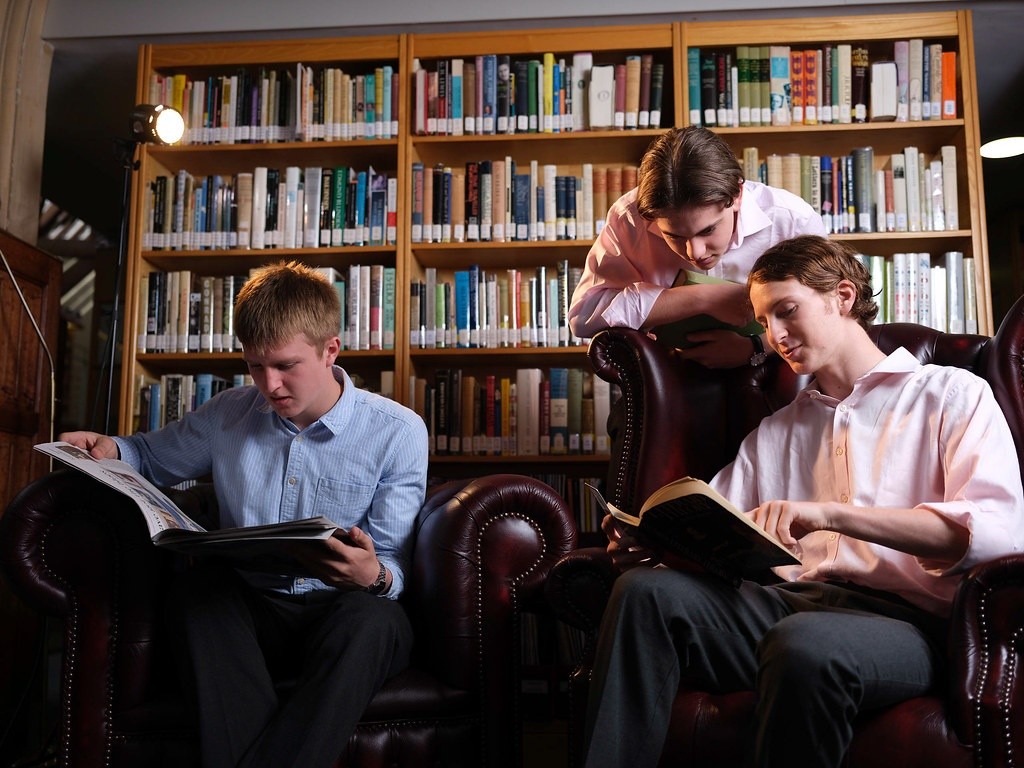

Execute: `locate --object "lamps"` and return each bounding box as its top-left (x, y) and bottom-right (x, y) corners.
top-left (114, 103), bottom-right (186, 171)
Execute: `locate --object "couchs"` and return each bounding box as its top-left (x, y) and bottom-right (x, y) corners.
top-left (0, 296), bottom-right (1024, 768)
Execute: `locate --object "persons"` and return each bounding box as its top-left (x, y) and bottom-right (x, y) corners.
top-left (59, 258), bottom-right (429, 768)
top-left (567, 126), bottom-right (832, 368)
top-left (578, 236), bottom-right (1024, 768)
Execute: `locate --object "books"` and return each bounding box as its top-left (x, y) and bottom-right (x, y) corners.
top-left (33, 442), bottom-right (349, 545)
top-left (133, 40), bottom-right (986, 534)
top-left (607, 475), bottom-right (802, 583)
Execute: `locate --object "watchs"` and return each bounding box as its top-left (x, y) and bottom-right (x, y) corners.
top-left (747, 332), bottom-right (767, 370)
top-left (361, 561), bottom-right (386, 595)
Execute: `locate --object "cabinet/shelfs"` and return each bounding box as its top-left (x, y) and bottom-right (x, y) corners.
top-left (118, 9), bottom-right (994, 551)
